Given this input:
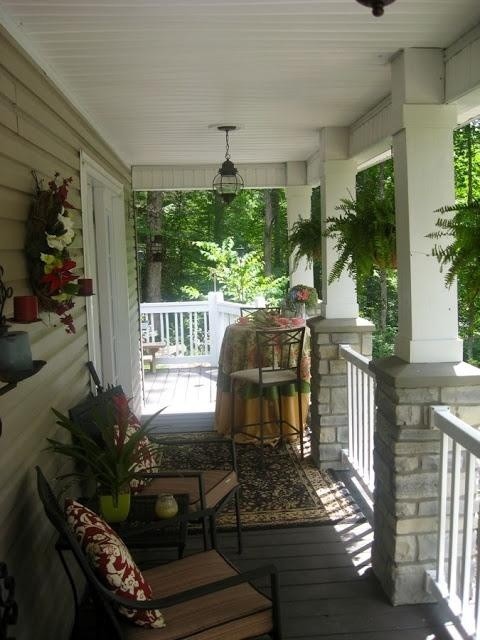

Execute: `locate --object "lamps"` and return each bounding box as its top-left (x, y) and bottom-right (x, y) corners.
top-left (213, 125), bottom-right (245, 208)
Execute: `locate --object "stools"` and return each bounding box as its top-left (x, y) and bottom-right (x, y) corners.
top-left (143, 354), bottom-right (153, 363)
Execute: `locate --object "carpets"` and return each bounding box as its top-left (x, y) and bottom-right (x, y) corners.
top-left (113, 430), bottom-right (367, 534)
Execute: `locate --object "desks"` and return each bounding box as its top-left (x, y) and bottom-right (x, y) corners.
top-left (212, 317), bottom-right (308, 446)
top-left (143, 341), bottom-right (166, 373)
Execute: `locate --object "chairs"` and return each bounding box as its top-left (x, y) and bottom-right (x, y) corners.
top-left (240, 308), bottom-right (280, 321)
top-left (228, 326), bottom-right (306, 458)
top-left (36, 387), bottom-right (281, 640)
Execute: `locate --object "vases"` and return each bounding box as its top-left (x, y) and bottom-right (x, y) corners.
top-left (294, 302), bottom-right (305, 319)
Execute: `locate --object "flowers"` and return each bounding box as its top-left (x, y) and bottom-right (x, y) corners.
top-left (286, 284), bottom-right (318, 312)
top-left (22, 167), bottom-right (84, 336)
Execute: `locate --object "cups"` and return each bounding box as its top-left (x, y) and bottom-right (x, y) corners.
top-left (278, 318), bottom-right (305, 326)
top-left (13, 296), bottom-right (38, 321)
top-left (78, 279), bottom-right (93, 295)
top-left (237, 317), bottom-right (249, 324)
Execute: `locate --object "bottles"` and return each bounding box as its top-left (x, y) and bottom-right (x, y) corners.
top-left (155, 493), bottom-right (178, 517)
